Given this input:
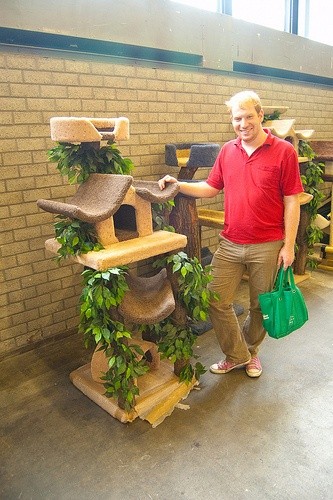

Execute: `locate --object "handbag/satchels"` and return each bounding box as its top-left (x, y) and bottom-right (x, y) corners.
top-left (257, 265), bottom-right (309, 339)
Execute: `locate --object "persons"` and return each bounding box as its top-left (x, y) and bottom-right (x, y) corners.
top-left (157, 88), bottom-right (305, 378)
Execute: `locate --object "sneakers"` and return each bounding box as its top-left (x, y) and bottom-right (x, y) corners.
top-left (210, 354), bottom-right (251, 373)
top-left (245, 357), bottom-right (263, 377)
